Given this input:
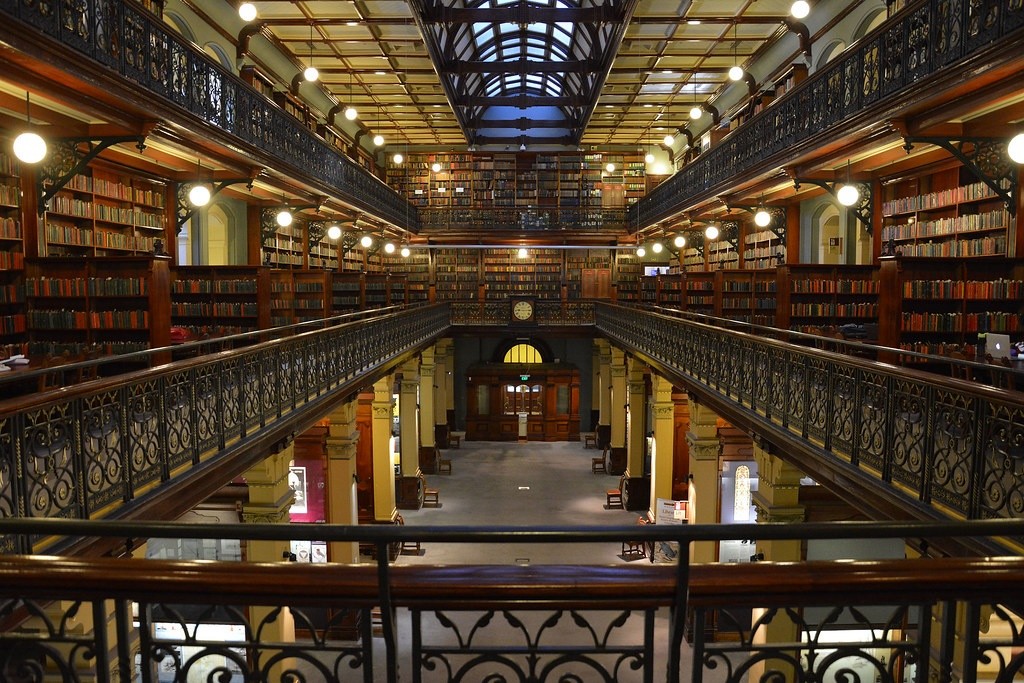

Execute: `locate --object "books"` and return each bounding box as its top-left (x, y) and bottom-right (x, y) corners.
top-left (333, 281), bottom-right (404, 323)
top-left (172, 278), bottom-right (258, 341)
top-left (790, 174), bottom-right (1024, 362)
top-left (0, 151), bottom-right (166, 366)
top-left (433, 249), bottom-right (611, 315)
top-left (387, 154), bottom-right (645, 225)
top-left (271, 281), bottom-right (324, 332)
top-left (265, 222), bottom-right (382, 272)
top-left (617, 228), bottom-right (785, 341)
top-left (385, 249), bottom-right (429, 299)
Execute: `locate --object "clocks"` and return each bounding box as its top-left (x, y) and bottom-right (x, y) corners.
top-left (510, 297), bottom-right (538, 327)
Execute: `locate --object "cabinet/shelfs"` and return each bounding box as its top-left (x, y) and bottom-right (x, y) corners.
top-left (0, 151), bottom-right (1024, 373)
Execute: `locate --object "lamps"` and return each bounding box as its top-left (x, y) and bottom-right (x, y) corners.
top-left (0, 0), bottom-right (1024, 256)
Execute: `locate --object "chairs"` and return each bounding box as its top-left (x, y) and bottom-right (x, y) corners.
top-left (25, 348), bottom-right (71, 440)
top-left (607, 475), bottom-right (626, 509)
top-left (448, 427), bottom-right (461, 449)
top-left (985, 354), bottom-right (1024, 450)
top-left (397, 515), bottom-right (420, 555)
top-left (591, 445), bottom-right (608, 474)
top-left (949, 351), bottom-right (971, 381)
top-left (420, 474), bottom-right (438, 507)
top-left (836, 333), bottom-right (848, 356)
top-left (622, 516), bottom-right (650, 560)
top-left (73, 344), bottom-right (105, 385)
top-left (585, 425), bottom-right (598, 448)
top-left (815, 329), bottom-right (824, 350)
top-left (437, 448), bottom-right (452, 475)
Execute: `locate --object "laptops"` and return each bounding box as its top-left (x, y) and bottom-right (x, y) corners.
top-left (986, 334), bottom-right (1017, 360)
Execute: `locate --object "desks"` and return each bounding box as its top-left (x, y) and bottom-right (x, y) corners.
top-left (954, 354), bottom-right (1024, 392)
top-left (0, 357), bottom-right (79, 404)
top-left (822, 332), bottom-right (879, 361)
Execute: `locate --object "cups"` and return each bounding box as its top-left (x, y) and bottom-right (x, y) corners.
top-left (977, 333), bottom-right (985, 357)
